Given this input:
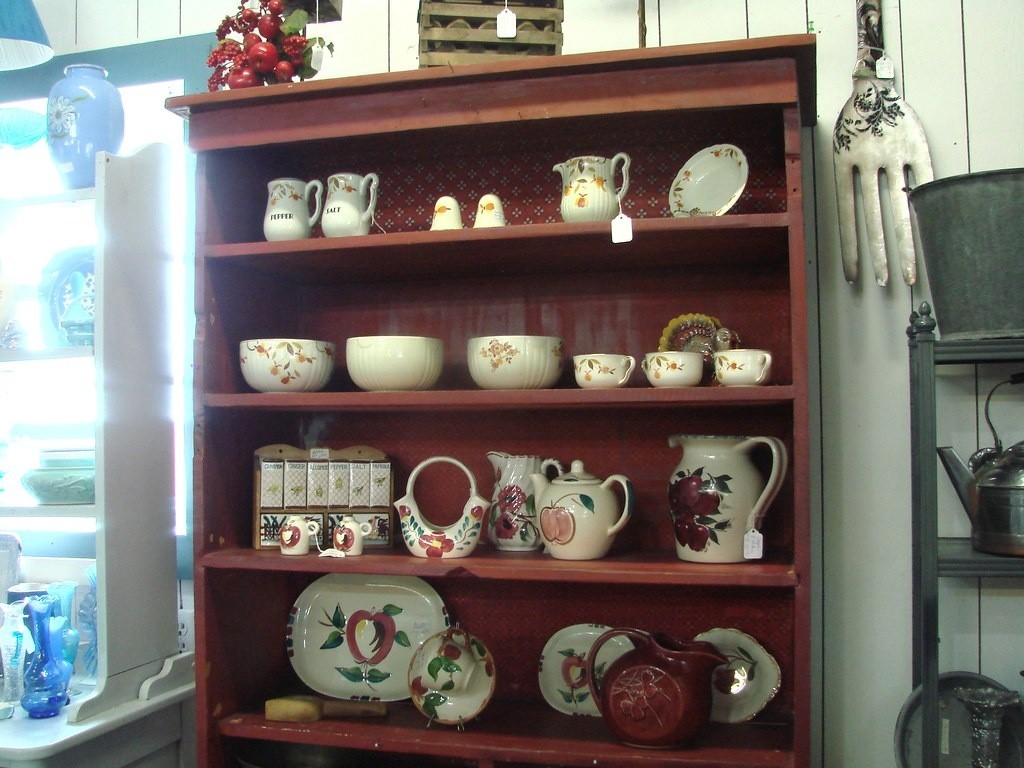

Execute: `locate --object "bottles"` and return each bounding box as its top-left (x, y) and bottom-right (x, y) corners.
top-left (279, 514), bottom-right (319, 556)
top-left (1, 582), bottom-right (81, 717)
top-left (44, 63), bottom-right (123, 189)
top-left (952, 686), bottom-right (1019, 768)
top-left (332, 513), bottom-right (371, 558)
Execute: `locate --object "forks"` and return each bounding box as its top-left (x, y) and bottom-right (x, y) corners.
top-left (833, 0), bottom-right (934, 285)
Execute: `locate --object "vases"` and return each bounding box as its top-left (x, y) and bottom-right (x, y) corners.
top-left (0, 580), bottom-right (78, 720)
top-left (46, 63), bottom-right (124, 188)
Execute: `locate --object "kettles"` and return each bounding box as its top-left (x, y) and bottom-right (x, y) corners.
top-left (934, 371), bottom-right (1024, 558)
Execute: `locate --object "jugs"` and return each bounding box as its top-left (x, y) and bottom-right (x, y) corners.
top-left (667, 434), bottom-right (787, 563)
top-left (262, 178), bottom-right (322, 242)
top-left (487, 450), bottom-right (561, 553)
top-left (552, 152), bottom-right (631, 222)
top-left (321, 173), bottom-right (381, 237)
top-left (583, 626), bottom-right (734, 750)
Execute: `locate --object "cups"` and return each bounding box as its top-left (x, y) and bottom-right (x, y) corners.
top-left (430, 195), bottom-right (462, 230)
top-left (473, 193), bottom-right (506, 227)
top-left (572, 348), bottom-right (773, 387)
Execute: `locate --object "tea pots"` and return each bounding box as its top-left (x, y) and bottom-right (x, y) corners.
top-left (530, 457), bottom-right (631, 562)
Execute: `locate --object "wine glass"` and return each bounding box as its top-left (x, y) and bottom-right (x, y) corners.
top-left (0, 107), bottom-right (44, 201)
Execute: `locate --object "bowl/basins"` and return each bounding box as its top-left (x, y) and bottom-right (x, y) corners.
top-left (238, 339), bottom-right (339, 393)
top-left (345, 337), bottom-right (444, 391)
top-left (466, 335), bottom-right (567, 388)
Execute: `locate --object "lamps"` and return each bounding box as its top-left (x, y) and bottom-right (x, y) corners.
top-left (0, 0), bottom-right (53, 71)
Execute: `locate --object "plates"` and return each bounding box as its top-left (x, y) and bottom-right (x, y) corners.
top-left (668, 143), bottom-right (749, 218)
top-left (287, 573), bottom-right (784, 722)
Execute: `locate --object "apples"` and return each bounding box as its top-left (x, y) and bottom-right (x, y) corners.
top-left (228, 0), bottom-right (293, 89)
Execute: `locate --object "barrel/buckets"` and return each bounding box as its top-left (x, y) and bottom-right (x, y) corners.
top-left (901, 165), bottom-right (1024, 341)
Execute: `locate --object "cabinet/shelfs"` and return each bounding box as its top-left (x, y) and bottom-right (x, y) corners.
top-left (905, 299), bottom-right (1024, 767)
top-left (163, 35), bottom-right (817, 768)
top-left (0, 138), bottom-right (197, 767)
